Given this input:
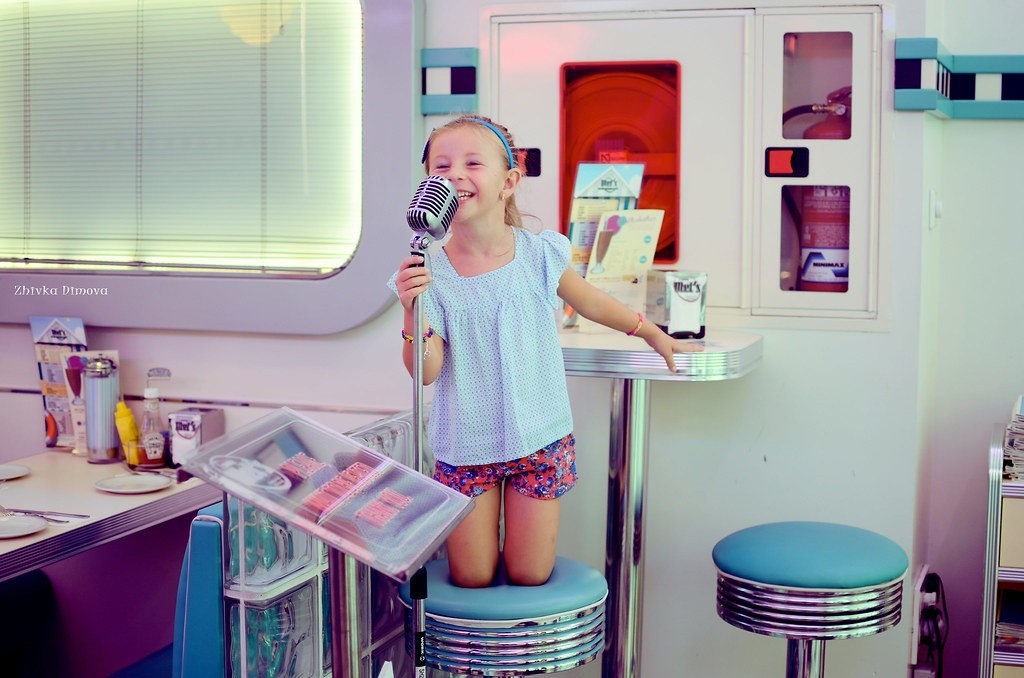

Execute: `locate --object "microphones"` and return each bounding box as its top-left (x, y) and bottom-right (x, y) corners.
top-left (406, 175), bottom-right (459, 254)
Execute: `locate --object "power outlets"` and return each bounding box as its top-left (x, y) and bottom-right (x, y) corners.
top-left (908, 563), bottom-right (932, 665)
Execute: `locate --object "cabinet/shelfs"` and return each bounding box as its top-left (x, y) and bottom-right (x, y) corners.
top-left (978, 427), bottom-right (1024, 678)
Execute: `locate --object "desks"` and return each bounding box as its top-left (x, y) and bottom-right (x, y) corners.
top-left (0, 441), bottom-right (226, 678)
top-left (561, 325), bottom-right (764, 678)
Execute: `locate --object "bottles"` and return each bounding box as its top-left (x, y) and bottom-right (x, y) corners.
top-left (115, 401), bottom-right (142, 465)
top-left (84, 358), bottom-right (118, 463)
top-left (139, 388), bottom-right (165, 464)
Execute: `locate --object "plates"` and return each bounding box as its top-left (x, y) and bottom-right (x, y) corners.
top-left (97, 474), bottom-right (171, 493)
top-left (0, 515), bottom-right (47, 539)
top-left (0, 464), bottom-right (30, 480)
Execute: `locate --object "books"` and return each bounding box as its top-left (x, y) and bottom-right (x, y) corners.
top-left (995, 395), bottom-right (1024, 650)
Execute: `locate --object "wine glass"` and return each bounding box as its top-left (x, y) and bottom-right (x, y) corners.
top-left (66, 369), bottom-right (85, 405)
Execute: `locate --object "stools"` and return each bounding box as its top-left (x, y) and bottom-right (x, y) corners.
top-left (712, 520), bottom-right (909, 678)
top-left (397, 553), bottom-right (609, 678)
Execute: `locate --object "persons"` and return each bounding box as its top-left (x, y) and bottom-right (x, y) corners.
top-left (387, 114), bottom-right (707, 588)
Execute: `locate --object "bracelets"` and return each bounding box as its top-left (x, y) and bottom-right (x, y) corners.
top-left (401, 325), bottom-right (434, 359)
top-left (627, 312), bottom-right (643, 336)
